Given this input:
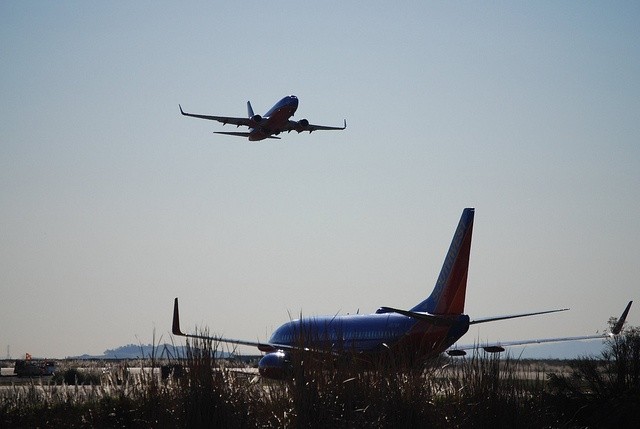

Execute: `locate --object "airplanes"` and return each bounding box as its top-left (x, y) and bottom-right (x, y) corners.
top-left (170, 207), bottom-right (633, 383)
top-left (177, 93), bottom-right (347, 141)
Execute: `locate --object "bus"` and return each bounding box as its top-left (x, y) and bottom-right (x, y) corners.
top-left (14, 357), bottom-right (56, 376)
